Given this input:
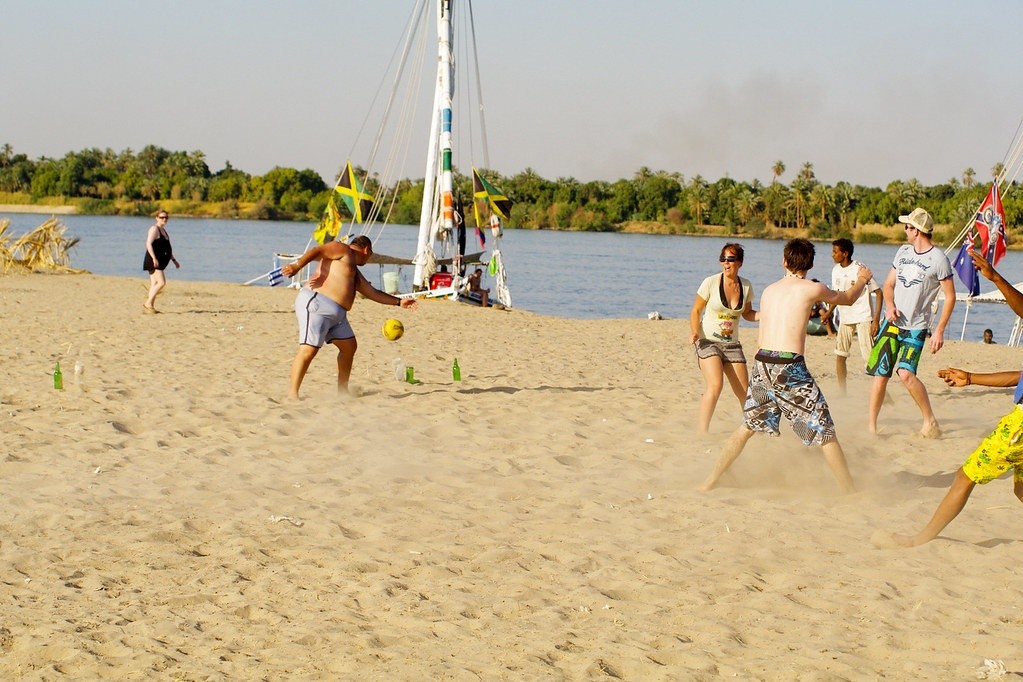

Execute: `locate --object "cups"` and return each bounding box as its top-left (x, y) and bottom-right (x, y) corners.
top-left (406, 366), bottom-right (414, 381)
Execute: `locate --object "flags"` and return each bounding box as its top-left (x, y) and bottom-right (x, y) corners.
top-left (473, 202), bottom-right (487, 247)
top-left (336, 162), bottom-right (376, 224)
top-left (975, 185), bottom-right (1007, 267)
top-left (473, 169), bottom-right (513, 222)
top-left (953, 227), bottom-right (981, 297)
top-left (313, 197), bottom-right (342, 245)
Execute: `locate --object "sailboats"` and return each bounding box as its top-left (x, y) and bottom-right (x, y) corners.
top-left (240, 0), bottom-right (513, 310)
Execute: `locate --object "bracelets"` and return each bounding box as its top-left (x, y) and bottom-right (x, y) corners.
top-left (966, 372), bottom-right (971, 384)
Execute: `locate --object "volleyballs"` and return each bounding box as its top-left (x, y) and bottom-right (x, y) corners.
top-left (383, 318), bottom-right (404, 341)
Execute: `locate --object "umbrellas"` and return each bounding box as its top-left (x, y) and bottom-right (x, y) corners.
top-left (975, 281), bottom-right (1023, 347)
top-left (871, 285), bottom-right (967, 300)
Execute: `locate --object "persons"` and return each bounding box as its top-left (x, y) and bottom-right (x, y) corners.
top-left (979, 328), bottom-right (997, 344)
top-left (866, 207), bottom-right (958, 439)
top-left (468, 269), bottom-right (490, 307)
top-left (143, 210), bottom-right (180, 314)
top-left (892, 249), bottom-right (1023, 550)
top-left (282, 234), bottom-right (418, 399)
top-left (691, 244), bottom-right (760, 435)
top-left (818, 237), bottom-right (884, 399)
top-left (698, 239), bottom-right (874, 499)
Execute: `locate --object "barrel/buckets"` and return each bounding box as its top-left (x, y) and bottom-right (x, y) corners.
top-left (383, 272), bottom-right (399, 293)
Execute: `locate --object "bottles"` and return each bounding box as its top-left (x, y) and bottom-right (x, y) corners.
top-left (54, 361), bottom-right (64, 390)
top-left (453, 357), bottom-right (461, 382)
